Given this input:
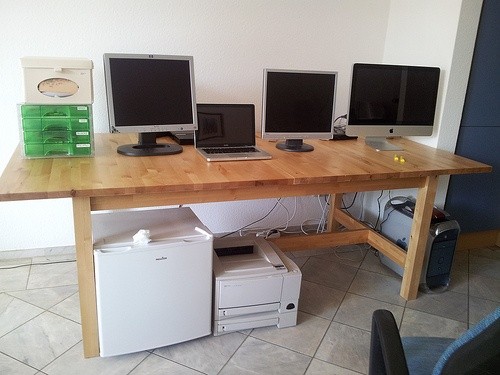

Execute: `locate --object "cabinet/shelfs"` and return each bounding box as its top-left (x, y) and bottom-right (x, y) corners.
top-left (92, 207), bottom-right (213, 357)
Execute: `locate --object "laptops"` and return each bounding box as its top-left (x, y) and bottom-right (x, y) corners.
top-left (194, 104), bottom-right (272, 161)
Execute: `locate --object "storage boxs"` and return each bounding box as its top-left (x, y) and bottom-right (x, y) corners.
top-left (16, 56), bottom-right (93, 160)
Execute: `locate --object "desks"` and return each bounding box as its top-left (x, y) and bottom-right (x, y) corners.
top-left (0, 131), bottom-right (493, 358)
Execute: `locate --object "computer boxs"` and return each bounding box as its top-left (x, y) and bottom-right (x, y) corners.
top-left (378, 195), bottom-right (460, 293)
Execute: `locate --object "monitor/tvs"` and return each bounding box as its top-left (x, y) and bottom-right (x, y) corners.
top-left (262, 68), bottom-right (338, 152)
top-left (102, 53), bottom-right (198, 156)
top-left (346, 63), bottom-right (440, 150)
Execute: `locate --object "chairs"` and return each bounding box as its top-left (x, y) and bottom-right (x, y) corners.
top-left (368, 309), bottom-right (500, 375)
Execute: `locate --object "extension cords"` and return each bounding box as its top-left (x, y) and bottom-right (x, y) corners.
top-left (256, 230), bottom-right (280, 239)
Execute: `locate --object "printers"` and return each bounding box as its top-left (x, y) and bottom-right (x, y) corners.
top-left (213, 235), bottom-right (301, 336)
